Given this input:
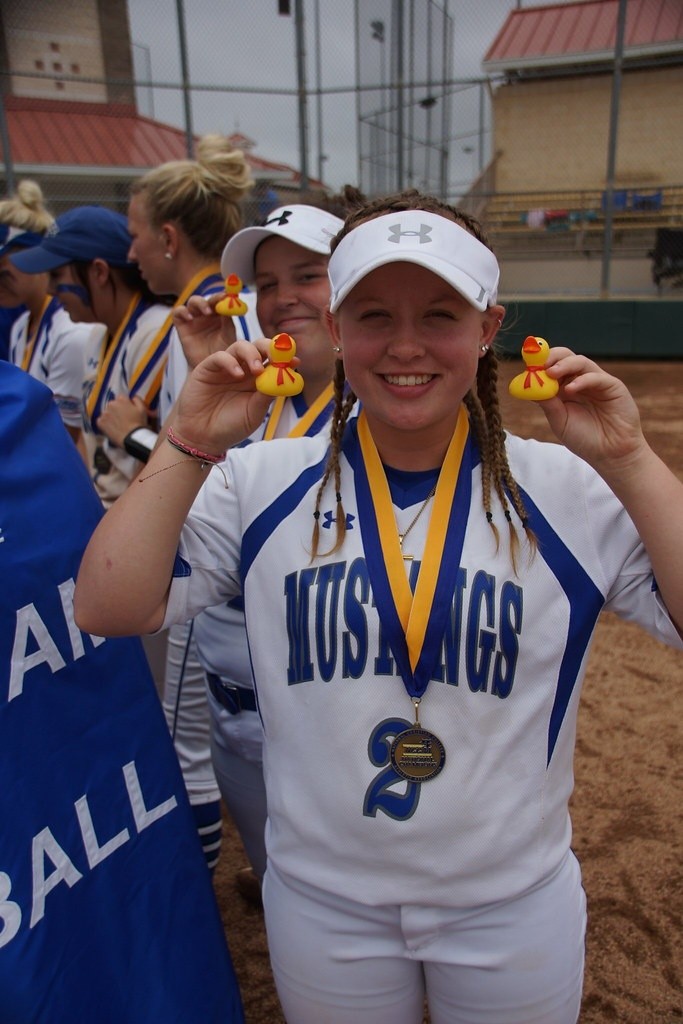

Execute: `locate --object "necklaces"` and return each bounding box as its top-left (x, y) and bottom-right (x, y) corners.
top-left (397, 483), bottom-right (439, 561)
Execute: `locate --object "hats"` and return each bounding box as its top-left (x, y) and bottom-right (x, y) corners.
top-left (0, 221), bottom-right (43, 259)
top-left (8, 204), bottom-right (138, 274)
top-left (219, 204), bottom-right (345, 288)
top-left (328, 209), bottom-right (503, 315)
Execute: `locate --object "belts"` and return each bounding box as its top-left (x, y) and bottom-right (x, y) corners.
top-left (207, 668), bottom-right (257, 712)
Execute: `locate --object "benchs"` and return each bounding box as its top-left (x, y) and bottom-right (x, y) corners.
top-left (477, 183), bottom-right (683, 237)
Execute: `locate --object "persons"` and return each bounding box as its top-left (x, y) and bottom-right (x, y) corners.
top-left (0, 131), bottom-right (367, 905)
top-left (72, 190), bottom-right (683, 1023)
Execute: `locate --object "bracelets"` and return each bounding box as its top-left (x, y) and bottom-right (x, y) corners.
top-left (139, 426), bottom-right (230, 490)
top-left (123, 425), bottom-right (161, 463)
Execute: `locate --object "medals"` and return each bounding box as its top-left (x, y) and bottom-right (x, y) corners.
top-left (389, 727), bottom-right (446, 783)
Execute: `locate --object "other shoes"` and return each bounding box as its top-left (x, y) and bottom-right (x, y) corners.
top-left (237, 864), bottom-right (267, 906)
